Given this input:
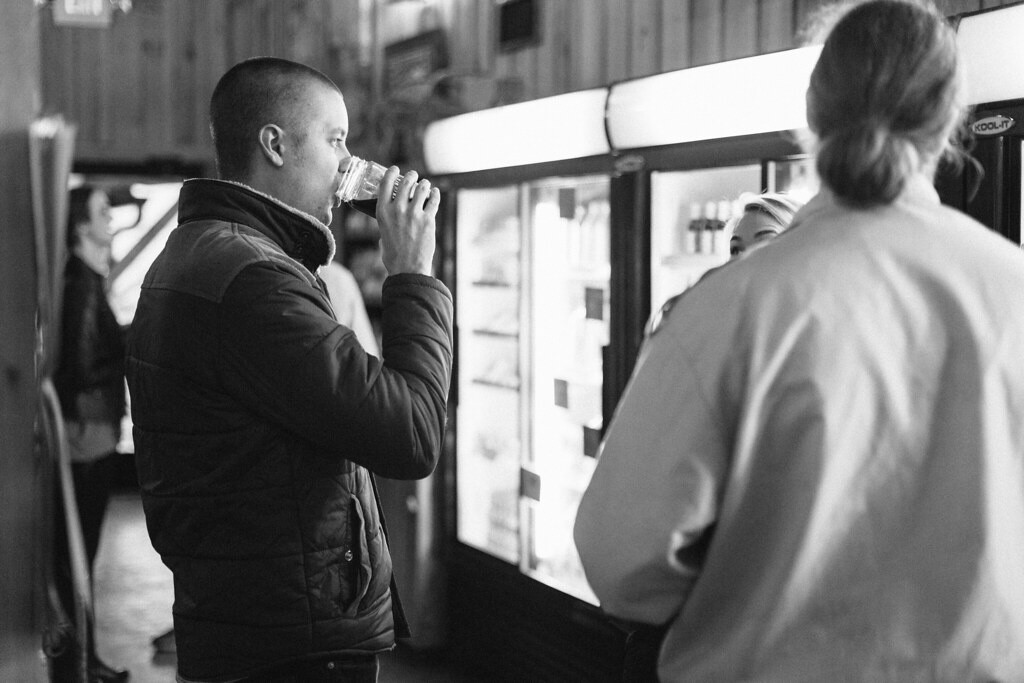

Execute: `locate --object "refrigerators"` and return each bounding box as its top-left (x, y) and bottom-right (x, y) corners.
top-left (422, 82), bottom-right (625, 681)
top-left (607, 47), bottom-right (832, 360)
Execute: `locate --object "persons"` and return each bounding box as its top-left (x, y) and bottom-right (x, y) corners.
top-left (125, 60), bottom-right (454, 683)
top-left (728, 191), bottom-right (801, 256)
top-left (44, 187), bottom-right (130, 683)
top-left (574, 0), bottom-right (1024, 683)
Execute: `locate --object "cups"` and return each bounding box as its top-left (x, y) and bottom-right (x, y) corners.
top-left (335, 154), bottom-right (432, 220)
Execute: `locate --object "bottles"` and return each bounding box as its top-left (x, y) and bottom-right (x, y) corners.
top-left (686, 200), bottom-right (731, 258)
